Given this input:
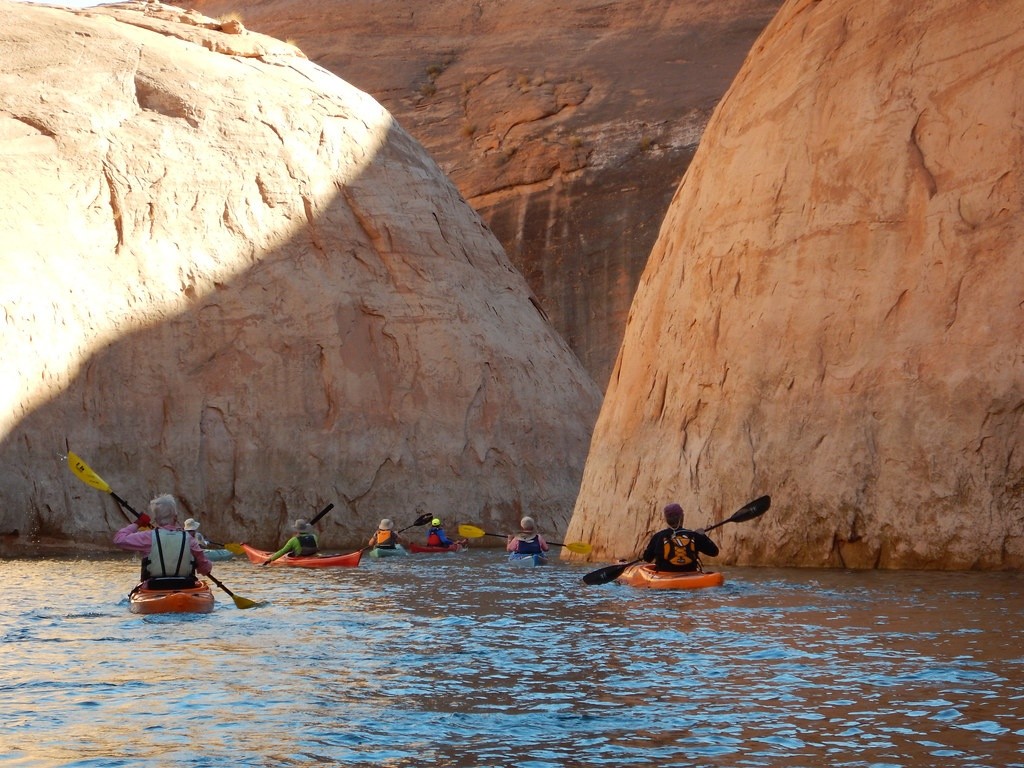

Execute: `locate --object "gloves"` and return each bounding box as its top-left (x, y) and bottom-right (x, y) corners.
top-left (135, 513), bottom-right (151, 527)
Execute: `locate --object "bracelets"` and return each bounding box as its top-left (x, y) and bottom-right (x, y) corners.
top-left (509, 540), bottom-right (511, 542)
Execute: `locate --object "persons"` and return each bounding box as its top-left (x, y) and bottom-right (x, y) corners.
top-left (506, 516), bottom-right (549, 556)
top-left (426, 518), bottom-right (468, 547)
top-left (267, 519), bottom-right (319, 563)
top-left (113, 494), bottom-right (212, 592)
top-left (369, 519), bottom-right (402, 550)
top-left (183, 519), bottom-right (210, 552)
top-left (644, 503), bottom-right (719, 572)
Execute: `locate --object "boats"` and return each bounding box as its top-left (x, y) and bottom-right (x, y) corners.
top-left (197, 548), bottom-right (233, 561)
top-left (244, 544), bottom-right (362, 568)
top-left (620, 562), bottom-right (722, 589)
top-left (370, 544), bottom-right (410, 559)
top-left (409, 540), bottom-right (466, 552)
top-left (509, 551), bottom-right (548, 567)
top-left (129, 573), bottom-right (215, 613)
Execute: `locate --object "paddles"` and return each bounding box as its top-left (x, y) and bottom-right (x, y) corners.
top-left (263, 503), bottom-right (335, 567)
top-left (140, 525), bottom-right (246, 555)
top-left (66, 450), bottom-right (255, 610)
top-left (582, 494), bottom-right (772, 586)
top-left (457, 524), bottom-right (592, 555)
top-left (362, 513), bottom-right (433, 551)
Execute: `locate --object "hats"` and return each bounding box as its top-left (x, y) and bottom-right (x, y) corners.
top-left (292, 519), bottom-right (311, 533)
top-left (431, 518), bottom-right (441, 526)
top-left (664, 504), bottom-right (682, 517)
top-left (184, 518), bottom-right (200, 530)
top-left (521, 517), bottom-right (534, 528)
top-left (379, 519), bottom-right (394, 530)
